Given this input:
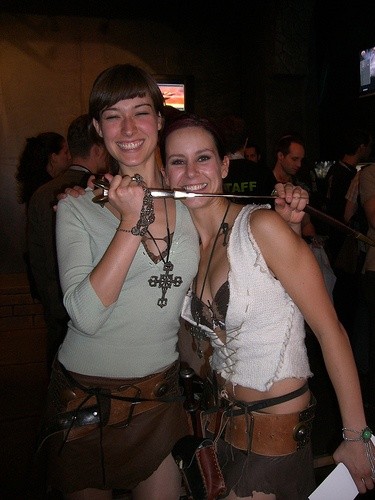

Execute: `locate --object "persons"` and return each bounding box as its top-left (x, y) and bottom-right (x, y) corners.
top-left (53, 116), bottom-right (375, 500)
top-left (210, 116), bottom-right (375, 374)
top-left (26, 113), bottom-right (111, 346)
top-left (43, 63), bottom-right (309, 500)
top-left (15, 130), bottom-right (72, 210)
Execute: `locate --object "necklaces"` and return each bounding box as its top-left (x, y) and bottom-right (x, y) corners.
top-left (148, 171), bottom-right (182, 308)
top-left (189, 200), bottom-right (233, 360)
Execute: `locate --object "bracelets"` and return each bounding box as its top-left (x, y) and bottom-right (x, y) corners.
top-left (340, 426), bottom-right (374, 482)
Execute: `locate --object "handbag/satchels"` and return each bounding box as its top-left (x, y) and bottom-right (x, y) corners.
top-left (172, 435), bottom-right (227, 500)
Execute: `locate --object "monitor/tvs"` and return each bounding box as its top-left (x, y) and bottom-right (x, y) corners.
top-left (154, 79), bottom-right (188, 114)
top-left (358, 43), bottom-right (375, 99)
